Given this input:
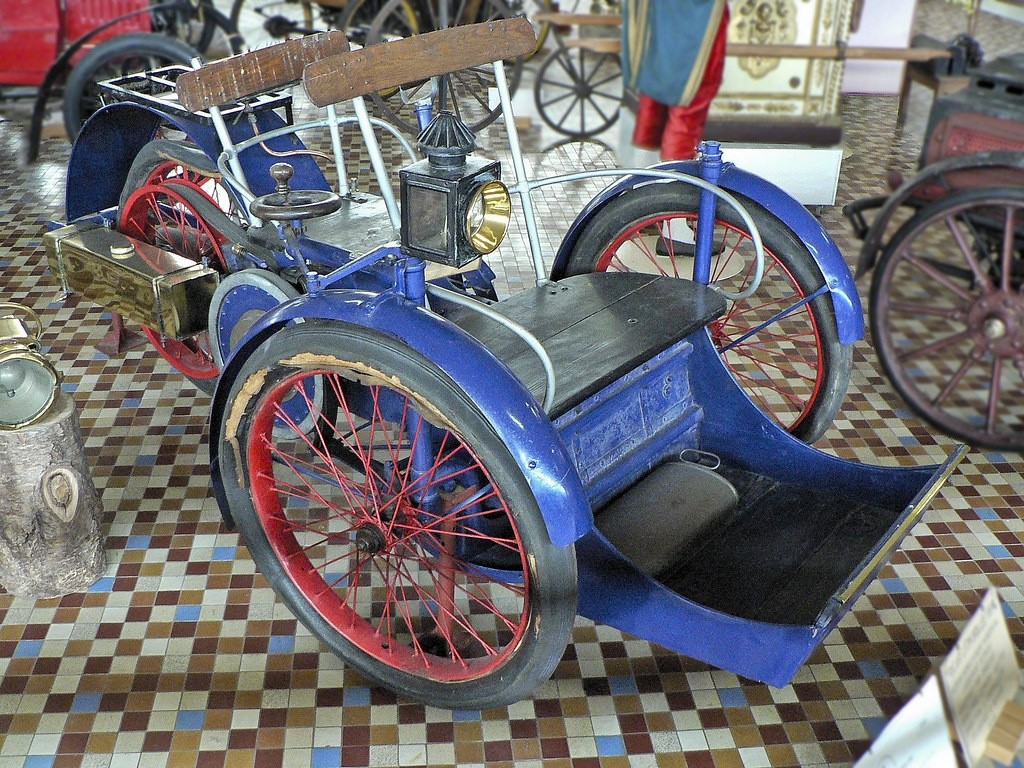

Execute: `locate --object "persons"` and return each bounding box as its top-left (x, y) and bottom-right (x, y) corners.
top-left (618, 0), bottom-right (730, 261)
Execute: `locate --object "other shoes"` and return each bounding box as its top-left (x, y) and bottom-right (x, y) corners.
top-left (656, 225), bottom-right (725, 257)
